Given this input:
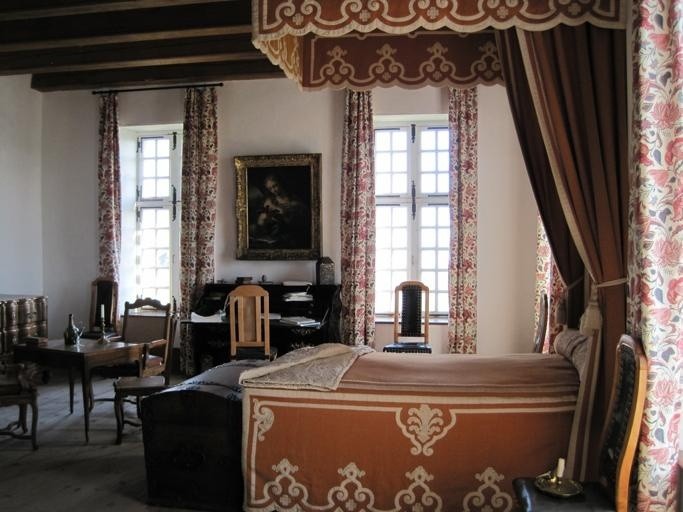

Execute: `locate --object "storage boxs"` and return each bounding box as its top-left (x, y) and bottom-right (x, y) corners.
top-left (140, 358), bottom-right (269, 511)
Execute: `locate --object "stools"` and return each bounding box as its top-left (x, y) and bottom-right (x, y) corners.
top-left (112, 376), bottom-right (166, 445)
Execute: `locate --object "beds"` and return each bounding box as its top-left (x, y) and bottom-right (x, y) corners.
top-left (240, 329), bottom-right (601, 512)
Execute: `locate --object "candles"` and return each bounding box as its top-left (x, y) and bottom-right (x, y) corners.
top-left (101, 304), bottom-right (104, 319)
top-left (555, 456), bottom-right (565, 478)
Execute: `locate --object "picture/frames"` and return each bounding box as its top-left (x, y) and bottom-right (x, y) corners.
top-left (232, 152), bottom-right (322, 262)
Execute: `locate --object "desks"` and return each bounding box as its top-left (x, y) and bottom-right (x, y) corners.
top-left (179, 284), bottom-right (343, 374)
top-left (14, 337), bottom-right (145, 444)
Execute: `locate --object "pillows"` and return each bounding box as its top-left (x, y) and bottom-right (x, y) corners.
top-left (570, 338), bottom-right (591, 382)
top-left (554, 330), bottom-right (588, 362)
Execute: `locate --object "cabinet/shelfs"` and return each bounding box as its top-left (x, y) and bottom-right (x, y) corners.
top-left (0, 294), bottom-right (48, 386)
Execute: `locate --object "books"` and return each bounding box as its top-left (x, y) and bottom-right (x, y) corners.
top-left (284, 292), bottom-right (313, 302)
top-left (279, 315), bottom-right (319, 328)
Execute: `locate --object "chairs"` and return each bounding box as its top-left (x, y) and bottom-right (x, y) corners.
top-left (513, 333), bottom-right (648, 512)
top-left (0, 373), bottom-right (39, 450)
top-left (82, 276), bottom-right (118, 340)
top-left (530, 294), bottom-right (548, 353)
top-left (228, 285), bottom-right (278, 362)
top-left (88, 298), bottom-right (171, 412)
top-left (383, 280), bottom-right (433, 354)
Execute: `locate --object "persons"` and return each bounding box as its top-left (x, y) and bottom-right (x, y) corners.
top-left (250, 178), bottom-right (303, 237)
